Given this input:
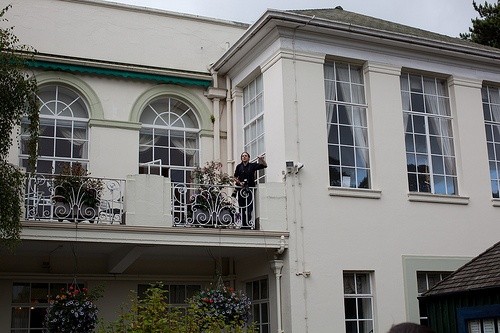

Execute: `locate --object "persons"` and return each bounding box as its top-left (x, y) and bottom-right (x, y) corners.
top-left (233, 152), bottom-right (267, 229)
